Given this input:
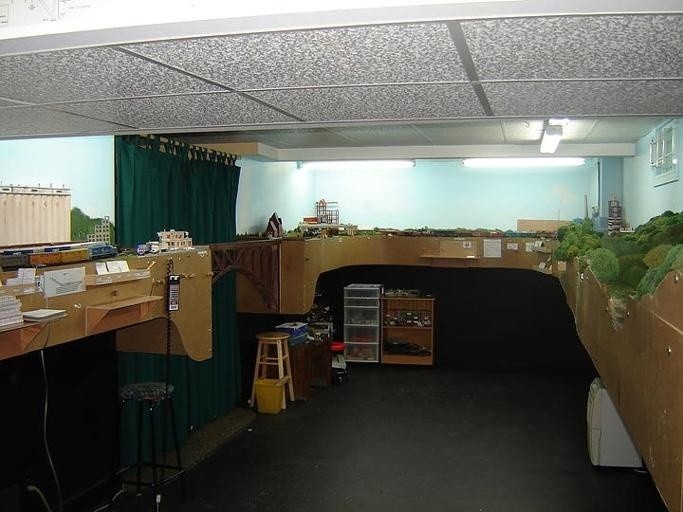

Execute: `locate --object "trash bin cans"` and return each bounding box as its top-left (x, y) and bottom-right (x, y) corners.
top-left (254, 379), bottom-right (283, 414)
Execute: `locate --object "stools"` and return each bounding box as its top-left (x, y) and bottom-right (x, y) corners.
top-left (247, 330), bottom-right (297, 411)
top-left (331, 341), bottom-right (346, 355)
top-left (116, 381), bottom-right (185, 501)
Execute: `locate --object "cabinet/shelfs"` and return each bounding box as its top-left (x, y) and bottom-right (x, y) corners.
top-left (344, 284), bottom-right (437, 365)
top-left (236, 235), bottom-right (683, 512)
top-left (0, 235), bottom-right (212, 512)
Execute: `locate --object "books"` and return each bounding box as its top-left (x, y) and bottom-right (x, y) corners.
top-left (21, 308), bottom-right (67, 322)
top-left (0, 294), bottom-right (23, 330)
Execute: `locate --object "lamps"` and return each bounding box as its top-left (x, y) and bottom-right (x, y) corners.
top-left (296, 125), bottom-right (585, 169)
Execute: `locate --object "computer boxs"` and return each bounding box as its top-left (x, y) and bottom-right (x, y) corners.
top-left (586, 378), bottom-right (643, 468)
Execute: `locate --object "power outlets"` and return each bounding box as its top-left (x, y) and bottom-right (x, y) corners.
top-left (15, 476), bottom-right (37, 497)
